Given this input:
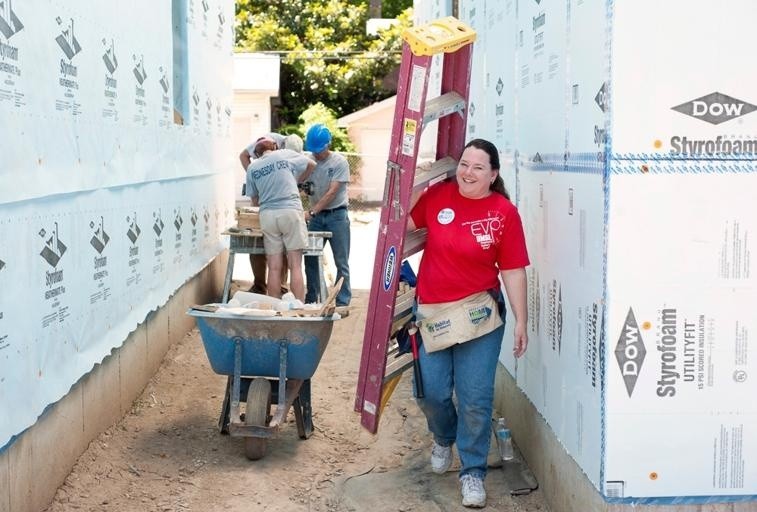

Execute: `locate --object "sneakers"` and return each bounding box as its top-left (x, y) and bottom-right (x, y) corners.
top-left (246, 282), bottom-right (266, 295)
top-left (458, 471), bottom-right (488, 507)
top-left (281, 284), bottom-right (350, 320)
top-left (430, 442), bottom-right (455, 475)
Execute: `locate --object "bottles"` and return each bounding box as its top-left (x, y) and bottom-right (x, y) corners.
top-left (495, 414), bottom-right (516, 465)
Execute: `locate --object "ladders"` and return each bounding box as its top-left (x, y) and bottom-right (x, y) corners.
top-left (353, 16), bottom-right (476, 434)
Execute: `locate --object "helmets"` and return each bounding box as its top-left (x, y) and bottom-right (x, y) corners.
top-left (303, 124), bottom-right (333, 153)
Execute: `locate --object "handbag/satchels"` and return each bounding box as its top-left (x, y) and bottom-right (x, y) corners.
top-left (415, 289), bottom-right (504, 356)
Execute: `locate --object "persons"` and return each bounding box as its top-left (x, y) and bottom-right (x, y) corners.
top-left (406, 137), bottom-right (530, 510)
top-left (244, 136), bottom-right (318, 301)
top-left (301, 124), bottom-right (352, 319)
top-left (237, 131), bottom-right (305, 294)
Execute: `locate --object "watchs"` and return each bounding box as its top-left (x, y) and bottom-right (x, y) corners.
top-left (308, 208), bottom-right (315, 219)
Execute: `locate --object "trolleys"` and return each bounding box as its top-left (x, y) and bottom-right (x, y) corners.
top-left (186, 296), bottom-right (341, 461)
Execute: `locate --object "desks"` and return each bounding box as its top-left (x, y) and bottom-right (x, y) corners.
top-left (220, 227), bottom-right (333, 305)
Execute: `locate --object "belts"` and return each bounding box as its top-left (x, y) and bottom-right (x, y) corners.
top-left (319, 204), bottom-right (348, 214)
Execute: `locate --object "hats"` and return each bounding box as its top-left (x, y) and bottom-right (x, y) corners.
top-left (253, 135), bottom-right (277, 157)
top-left (284, 133), bottom-right (303, 155)
